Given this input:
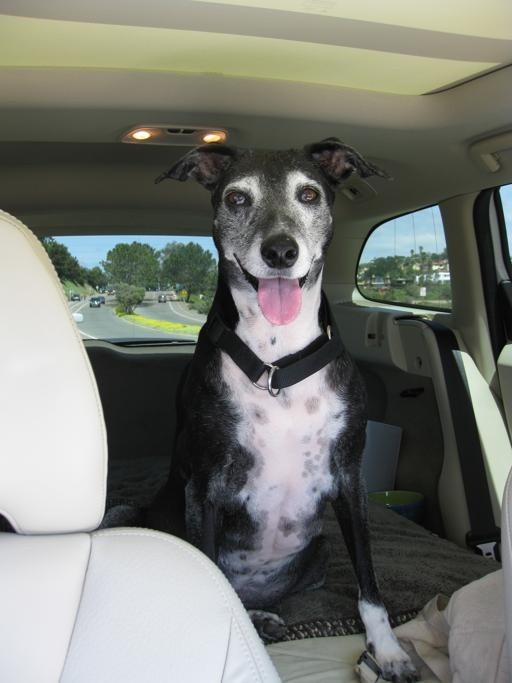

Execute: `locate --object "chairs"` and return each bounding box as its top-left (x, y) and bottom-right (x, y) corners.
top-left (0, 207), bottom-right (282, 681)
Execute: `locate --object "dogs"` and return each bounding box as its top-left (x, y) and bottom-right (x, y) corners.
top-left (102, 136), bottom-right (421, 683)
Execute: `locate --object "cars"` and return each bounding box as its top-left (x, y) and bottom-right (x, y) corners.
top-left (71, 286), bottom-right (114, 307)
top-left (158, 294), bottom-right (167, 303)
